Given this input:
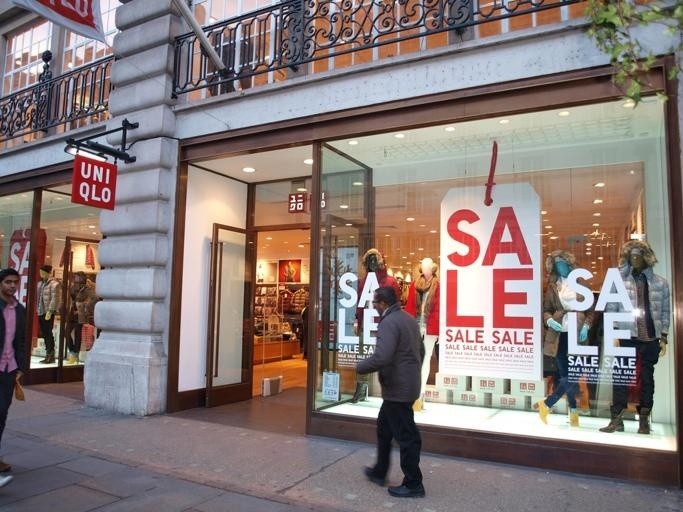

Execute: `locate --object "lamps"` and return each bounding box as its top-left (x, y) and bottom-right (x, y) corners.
top-left (63, 117), bottom-right (140, 166)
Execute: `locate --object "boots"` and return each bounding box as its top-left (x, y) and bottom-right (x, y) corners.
top-left (349, 381), bottom-right (369, 403)
top-left (599, 405), bottom-right (627, 434)
top-left (636, 406), bottom-right (652, 434)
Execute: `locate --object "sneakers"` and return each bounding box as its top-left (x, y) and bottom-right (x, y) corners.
top-left (0, 457), bottom-right (11, 473)
top-left (412, 398), bottom-right (424, 411)
top-left (0, 476), bottom-right (13, 490)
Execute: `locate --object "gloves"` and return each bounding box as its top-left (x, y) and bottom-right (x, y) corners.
top-left (577, 324), bottom-right (589, 344)
top-left (548, 316), bottom-right (562, 332)
top-left (351, 318), bottom-right (362, 336)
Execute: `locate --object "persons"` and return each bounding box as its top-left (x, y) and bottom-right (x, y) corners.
top-left (63, 272), bottom-right (94, 364)
top-left (36, 264), bottom-right (61, 364)
top-left (404, 257), bottom-right (439, 412)
top-left (0, 268), bottom-right (26, 489)
top-left (283, 261), bottom-right (297, 281)
top-left (536, 249), bottom-right (599, 428)
top-left (301, 301), bottom-right (310, 359)
top-left (355, 286), bottom-right (426, 498)
top-left (599, 239), bottom-right (671, 433)
top-left (349, 248), bottom-right (402, 404)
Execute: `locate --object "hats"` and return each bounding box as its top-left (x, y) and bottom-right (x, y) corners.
top-left (40, 264), bottom-right (87, 279)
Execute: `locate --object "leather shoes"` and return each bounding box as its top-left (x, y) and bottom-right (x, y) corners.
top-left (387, 481), bottom-right (427, 499)
top-left (361, 463), bottom-right (388, 487)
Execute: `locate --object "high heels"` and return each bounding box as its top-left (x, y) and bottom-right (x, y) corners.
top-left (534, 400), bottom-right (550, 426)
top-left (568, 408), bottom-right (581, 427)
top-left (38, 352), bottom-right (80, 365)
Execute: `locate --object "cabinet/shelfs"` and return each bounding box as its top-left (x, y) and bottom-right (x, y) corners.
top-left (254, 283), bottom-right (311, 360)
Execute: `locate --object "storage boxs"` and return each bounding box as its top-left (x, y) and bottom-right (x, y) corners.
top-left (426, 373), bottom-right (568, 414)
